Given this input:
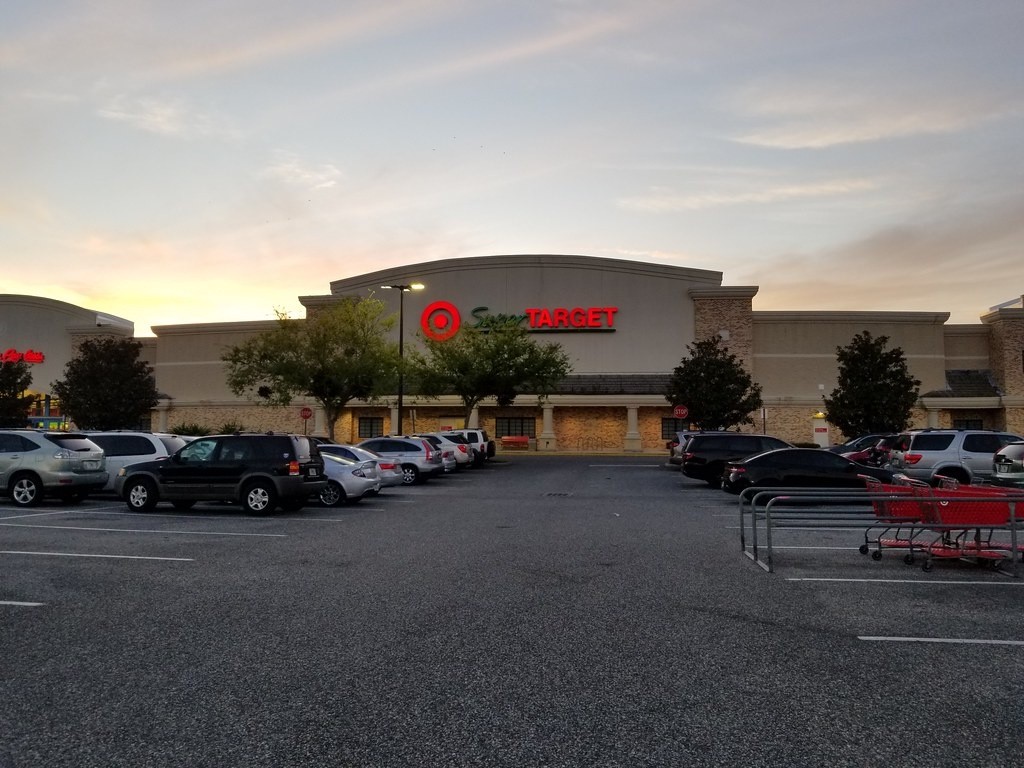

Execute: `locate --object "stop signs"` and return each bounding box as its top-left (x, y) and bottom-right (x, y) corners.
top-left (674, 405), bottom-right (689, 419)
top-left (301, 408), bottom-right (312, 419)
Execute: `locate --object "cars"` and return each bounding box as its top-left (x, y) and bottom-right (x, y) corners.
top-left (435, 447), bottom-right (456, 478)
top-left (319, 452), bottom-right (381, 508)
top-left (410, 433), bottom-right (475, 472)
top-left (180, 436), bottom-right (243, 460)
top-left (316, 445), bottom-right (405, 497)
top-left (992, 441), bottom-right (1024, 489)
top-left (720, 447), bottom-right (930, 506)
top-left (828, 435), bottom-right (899, 468)
top-left (310, 436), bottom-right (339, 445)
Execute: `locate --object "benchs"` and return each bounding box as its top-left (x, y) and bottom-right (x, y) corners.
top-left (500, 436), bottom-right (529, 450)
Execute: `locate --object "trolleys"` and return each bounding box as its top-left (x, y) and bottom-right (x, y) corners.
top-left (892, 473), bottom-right (1024, 573)
top-left (933, 474), bottom-right (1024, 568)
top-left (856, 473), bottom-right (953, 561)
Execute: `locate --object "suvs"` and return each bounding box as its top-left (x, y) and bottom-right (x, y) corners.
top-left (440, 427), bottom-right (496, 469)
top-left (115, 431), bottom-right (329, 518)
top-left (0, 427), bottom-right (111, 508)
top-left (669, 431), bottom-right (740, 465)
top-left (885, 426), bottom-right (1024, 488)
top-left (681, 432), bottom-right (798, 489)
top-left (81, 429), bottom-right (188, 497)
top-left (356, 434), bottom-right (444, 487)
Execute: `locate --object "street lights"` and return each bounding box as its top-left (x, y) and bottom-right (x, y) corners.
top-left (379, 283), bottom-right (427, 434)
